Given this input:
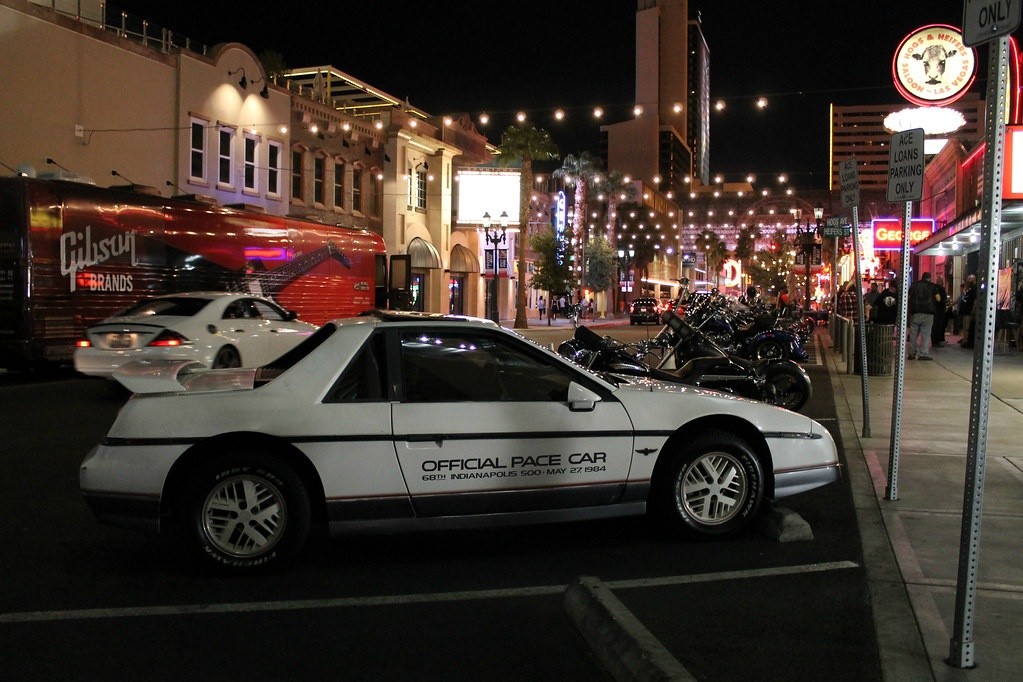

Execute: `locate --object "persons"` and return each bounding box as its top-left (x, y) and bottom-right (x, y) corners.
top-left (536, 295), bottom-right (596, 322)
top-left (708, 272), bottom-right (978, 361)
top-left (619, 299), bottom-right (630, 318)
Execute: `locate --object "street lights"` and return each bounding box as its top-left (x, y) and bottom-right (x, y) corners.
top-left (790, 201), bottom-right (824, 310)
top-left (617, 247), bottom-right (635, 313)
top-left (483, 211), bottom-right (510, 324)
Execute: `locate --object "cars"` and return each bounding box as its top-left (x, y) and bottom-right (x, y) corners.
top-left (68, 292), bottom-right (319, 394)
top-left (78, 310), bottom-right (840, 593)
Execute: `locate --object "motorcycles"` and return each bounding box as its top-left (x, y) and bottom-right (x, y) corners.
top-left (557, 276), bottom-right (815, 412)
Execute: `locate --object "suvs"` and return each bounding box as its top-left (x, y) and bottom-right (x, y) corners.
top-left (629, 298), bottom-right (659, 325)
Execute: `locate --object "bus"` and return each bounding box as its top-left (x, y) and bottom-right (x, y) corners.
top-left (0, 175), bottom-right (414, 377)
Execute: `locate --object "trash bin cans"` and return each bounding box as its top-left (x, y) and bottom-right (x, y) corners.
top-left (853, 324), bottom-right (895, 376)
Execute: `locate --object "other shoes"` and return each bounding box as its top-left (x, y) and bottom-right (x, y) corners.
top-left (932, 342), bottom-right (944, 348)
top-left (960, 342), bottom-right (974, 348)
top-left (907, 357), bottom-right (915, 360)
top-left (918, 356), bottom-right (932, 360)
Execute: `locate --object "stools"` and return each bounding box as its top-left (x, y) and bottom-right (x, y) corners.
top-left (995, 321), bottom-right (1019, 355)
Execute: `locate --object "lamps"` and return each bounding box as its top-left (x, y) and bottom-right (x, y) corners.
top-left (303, 126), bottom-right (324, 139)
top-left (228, 67), bottom-right (247, 89)
top-left (414, 155), bottom-right (429, 171)
top-left (251, 77), bottom-right (270, 98)
top-left (352, 141), bottom-right (371, 156)
top-left (373, 150), bottom-right (391, 163)
top-left (329, 132), bottom-right (349, 148)
